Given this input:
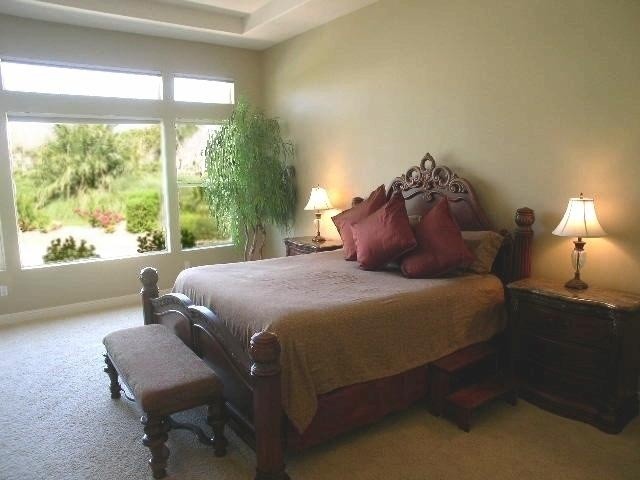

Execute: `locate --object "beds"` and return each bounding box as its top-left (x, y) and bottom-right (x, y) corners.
top-left (141, 151), bottom-right (535, 479)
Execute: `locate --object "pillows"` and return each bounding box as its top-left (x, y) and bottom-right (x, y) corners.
top-left (330, 183), bottom-right (502, 278)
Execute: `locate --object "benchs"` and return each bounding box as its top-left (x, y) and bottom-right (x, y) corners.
top-left (101, 321), bottom-right (230, 480)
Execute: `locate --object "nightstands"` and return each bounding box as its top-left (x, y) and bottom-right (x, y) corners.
top-left (504, 277), bottom-right (640, 435)
top-left (284, 237), bottom-right (342, 255)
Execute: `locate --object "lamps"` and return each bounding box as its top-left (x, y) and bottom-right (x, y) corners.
top-left (552, 193), bottom-right (608, 292)
top-left (303, 183), bottom-right (335, 242)
